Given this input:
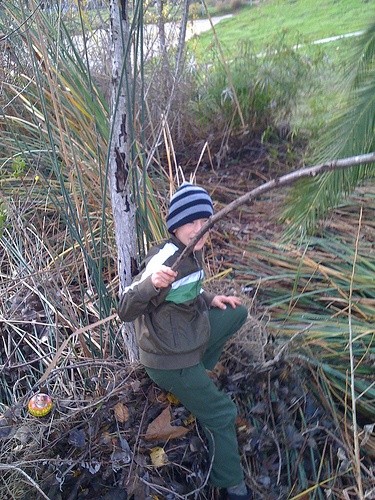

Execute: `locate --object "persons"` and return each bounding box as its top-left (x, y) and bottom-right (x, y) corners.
top-left (117, 181), bottom-right (263, 500)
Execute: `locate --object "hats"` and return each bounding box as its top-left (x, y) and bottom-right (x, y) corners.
top-left (165, 182), bottom-right (214, 234)
